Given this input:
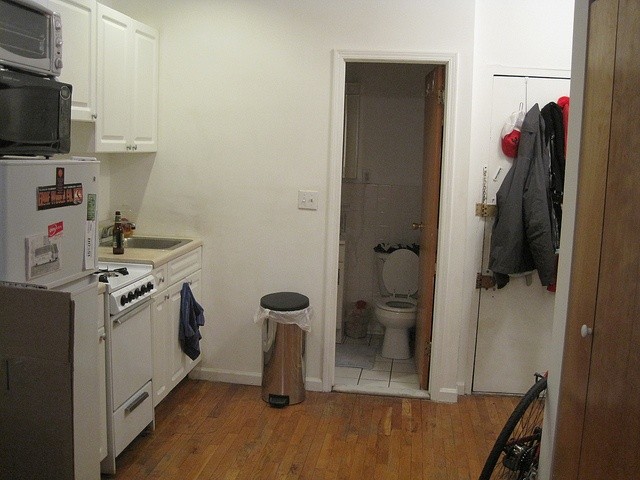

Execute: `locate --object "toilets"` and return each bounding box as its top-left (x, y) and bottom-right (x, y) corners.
top-left (375, 247), bottom-right (419, 361)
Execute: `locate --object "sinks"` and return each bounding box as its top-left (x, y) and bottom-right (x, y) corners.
top-left (99, 237), bottom-right (193, 251)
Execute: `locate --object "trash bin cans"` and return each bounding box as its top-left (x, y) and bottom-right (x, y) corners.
top-left (257, 292), bottom-right (311, 408)
top-left (345, 301), bottom-right (371, 338)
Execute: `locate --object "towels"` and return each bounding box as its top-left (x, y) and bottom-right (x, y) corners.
top-left (179, 282), bottom-right (206, 361)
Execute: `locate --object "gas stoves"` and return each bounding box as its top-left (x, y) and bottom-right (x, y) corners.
top-left (97, 263), bottom-right (152, 291)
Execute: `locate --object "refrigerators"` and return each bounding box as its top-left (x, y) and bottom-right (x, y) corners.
top-left (0, 159), bottom-right (102, 462)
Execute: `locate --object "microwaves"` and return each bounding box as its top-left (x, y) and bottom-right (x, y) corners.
top-left (0, 70), bottom-right (72, 159)
top-left (0, 0), bottom-right (63, 76)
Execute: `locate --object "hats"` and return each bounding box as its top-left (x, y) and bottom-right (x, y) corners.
top-left (501, 102), bottom-right (526, 158)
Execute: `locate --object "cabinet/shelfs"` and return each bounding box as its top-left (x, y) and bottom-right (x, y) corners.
top-left (167, 244), bottom-right (203, 392)
top-left (46, 0), bottom-right (96, 124)
top-left (152, 262), bottom-right (168, 408)
top-left (68, 1), bottom-right (160, 155)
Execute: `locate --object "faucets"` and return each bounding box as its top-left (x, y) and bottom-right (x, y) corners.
top-left (102, 222), bottom-right (136, 237)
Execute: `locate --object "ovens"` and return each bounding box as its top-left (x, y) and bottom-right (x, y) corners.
top-left (109, 273), bottom-right (157, 459)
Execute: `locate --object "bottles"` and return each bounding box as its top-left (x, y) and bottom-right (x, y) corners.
top-left (112, 211), bottom-right (125, 254)
